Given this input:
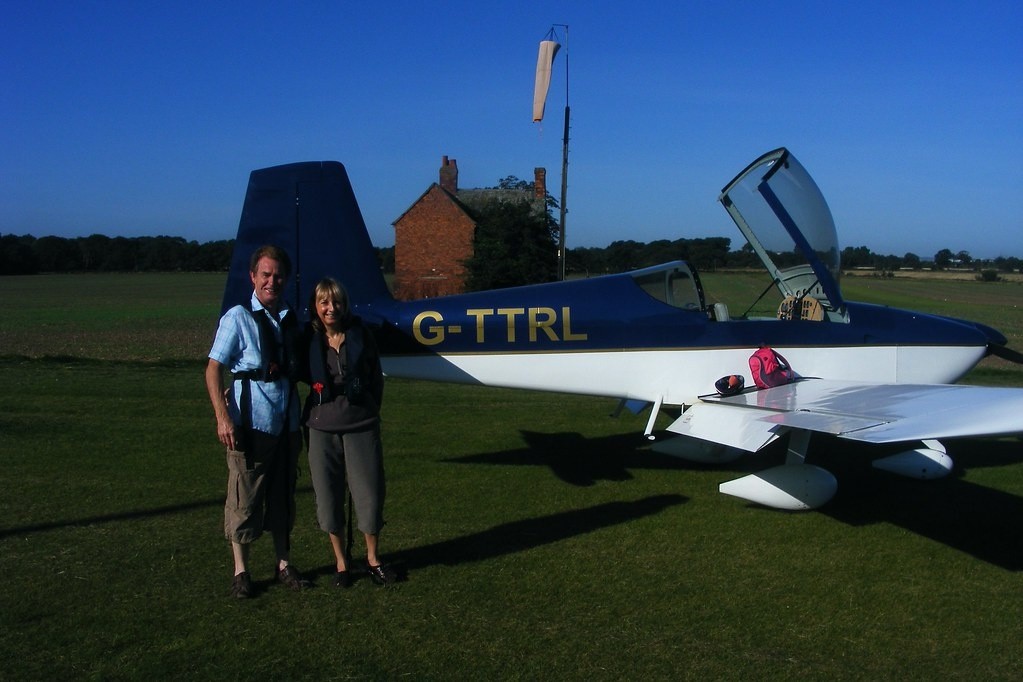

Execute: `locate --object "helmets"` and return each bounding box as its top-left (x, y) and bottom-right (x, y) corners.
top-left (715, 375), bottom-right (744, 397)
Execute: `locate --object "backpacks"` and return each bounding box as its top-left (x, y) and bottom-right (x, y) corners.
top-left (749, 348), bottom-right (794, 390)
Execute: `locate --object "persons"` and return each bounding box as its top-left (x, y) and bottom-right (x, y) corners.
top-left (205, 246), bottom-right (307, 597)
top-left (224, 279), bottom-right (397, 587)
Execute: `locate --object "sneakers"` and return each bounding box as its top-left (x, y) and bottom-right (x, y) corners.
top-left (365, 561), bottom-right (393, 586)
top-left (332, 569), bottom-right (348, 590)
top-left (231, 572), bottom-right (251, 600)
top-left (275, 564), bottom-right (306, 589)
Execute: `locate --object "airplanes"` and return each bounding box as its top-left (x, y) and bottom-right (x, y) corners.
top-left (217, 145), bottom-right (1023, 511)
